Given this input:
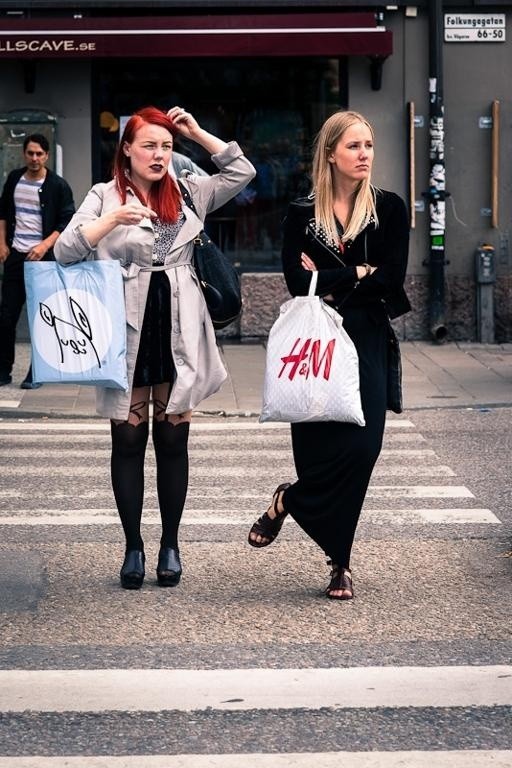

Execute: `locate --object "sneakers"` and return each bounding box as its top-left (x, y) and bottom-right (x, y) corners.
top-left (21, 379), bottom-right (42, 389)
top-left (1, 376), bottom-right (12, 387)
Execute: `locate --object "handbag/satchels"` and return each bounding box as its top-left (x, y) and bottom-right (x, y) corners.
top-left (24, 260), bottom-right (128, 393)
top-left (194, 230), bottom-right (242, 331)
top-left (259, 296), bottom-right (366, 427)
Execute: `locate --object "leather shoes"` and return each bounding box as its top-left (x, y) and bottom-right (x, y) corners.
top-left (120, 551), bottom-right (145, 589)
top-left (157, 546), bottom-right (181, 587)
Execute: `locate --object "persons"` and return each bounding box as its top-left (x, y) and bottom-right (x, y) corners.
top-left (53, 105), bottom-right (257, 588)
top-left (0, 133), bottom-right (76, 389)
top-left (184, 129), bottom-right (316, 266)
top-left (250, 109), bottom-right (412, 599)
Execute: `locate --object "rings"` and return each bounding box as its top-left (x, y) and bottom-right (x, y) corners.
top-left (134, 207), bottom-right (137, 215)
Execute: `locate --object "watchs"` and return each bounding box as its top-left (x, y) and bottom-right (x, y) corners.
top-left (361, 263), bottom-right (371, 277)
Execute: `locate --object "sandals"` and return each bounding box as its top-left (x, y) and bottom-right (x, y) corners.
top-left (248, 483), bottom-right (290, 548)
top-left (326, 560), bottom-right (354, 600)
